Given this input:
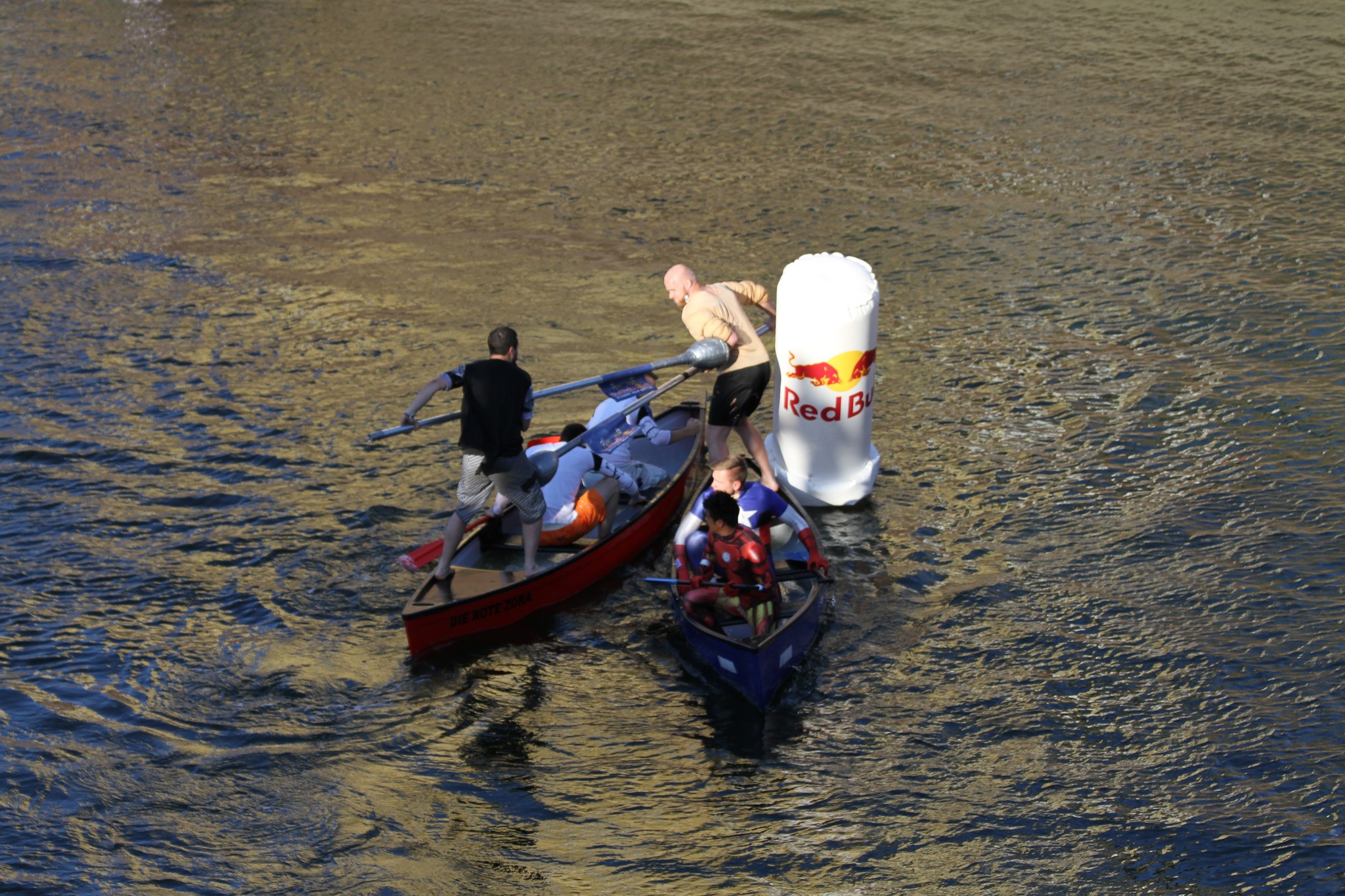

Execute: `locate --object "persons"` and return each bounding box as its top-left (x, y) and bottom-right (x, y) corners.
top-left (400, 325), bottom-right (546, 581)
top-left (663, 262), bottom-right (780, 493)
top-left (582, 371), bottom-right (701, 491)
top-left (484, 423), bottom-right (646, 548)
top-left (669, 453), bottom-right (828, 595)
top-left (677, 490), bottom-right (783, 644)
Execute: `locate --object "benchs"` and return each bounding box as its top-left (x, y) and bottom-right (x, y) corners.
top-left (719, 600), bottom-right (809, 627)
top-left (773, 560), bottom-right (817, 581)
top-left (575, 484), bottom-right (663, 505)
top-left (488, 533), bottom-right (600, 554)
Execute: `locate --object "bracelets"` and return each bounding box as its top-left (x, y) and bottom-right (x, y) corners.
top-left (403, 412), bottom-right (415, 419)
top-left (631, 493), bottom-right (641, 500)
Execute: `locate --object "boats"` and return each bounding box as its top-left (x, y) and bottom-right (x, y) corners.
top-left (670, 450), bottom-right (833, 712)
top-left (399, 386), bottom-right (711, 661)
